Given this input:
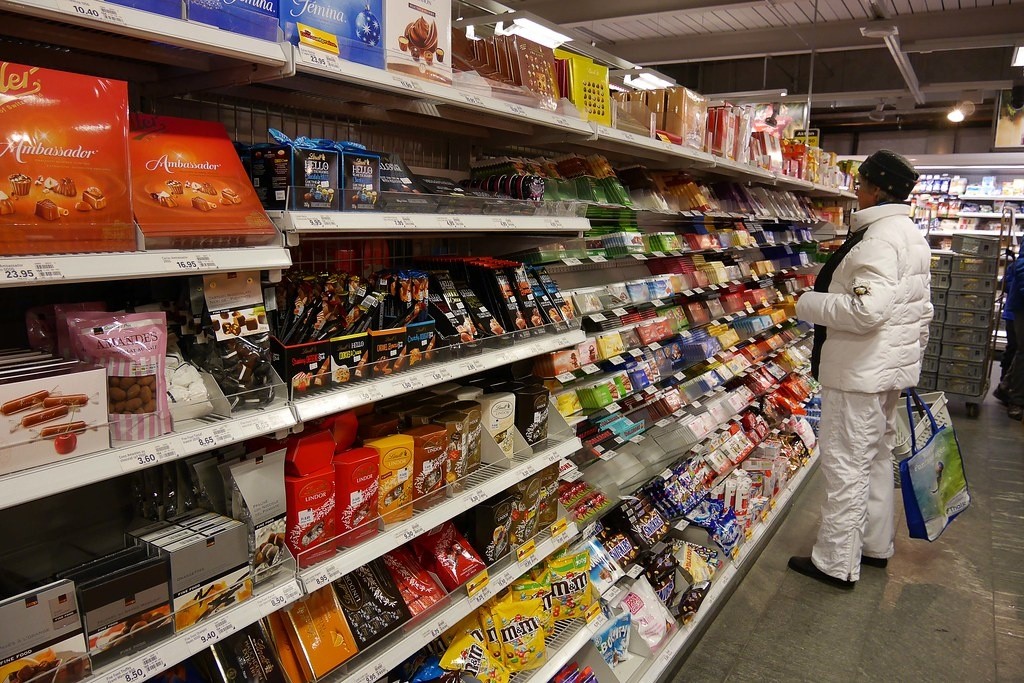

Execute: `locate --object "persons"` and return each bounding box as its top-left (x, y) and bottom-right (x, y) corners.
top-left (994, 235), bottom-right (1024, 421)
top-left (772, 150), bottom-right (934, 589)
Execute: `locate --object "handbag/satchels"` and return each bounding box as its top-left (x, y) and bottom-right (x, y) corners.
top-left (899, 387), bottom-right (971, 543)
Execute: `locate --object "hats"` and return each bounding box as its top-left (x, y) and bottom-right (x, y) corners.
top-left (858, 149), bottom-right (919, 200)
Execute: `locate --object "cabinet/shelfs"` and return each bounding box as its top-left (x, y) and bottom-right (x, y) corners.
top-left (955, 196), bottom-right (1024, 351)
top-left (0, 0), bottom-right (860, 682)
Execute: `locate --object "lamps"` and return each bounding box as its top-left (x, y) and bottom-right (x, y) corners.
top-left (869, 102), bottom-right (885, 122)
top-left (451, 9), bottom-right (574, 50)
top-left (702, 57), bottom-right (788, 102)
top-left (609, 68), bottom-right (676, 89)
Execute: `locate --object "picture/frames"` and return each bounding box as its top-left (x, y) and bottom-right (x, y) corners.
top-left (988, 90), bottom-right (1024, 153)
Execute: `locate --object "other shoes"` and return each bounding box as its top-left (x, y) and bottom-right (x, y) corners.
top-left (993, 383), bottom-right (1009, 404)
top-left (860, 555), bottom-right (887, 568)
top-left (788, 556), bottom-right (855, 590)
top-left (1007, 403), bottom-right (1023, 421)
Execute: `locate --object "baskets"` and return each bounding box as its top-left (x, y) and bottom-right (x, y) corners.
top-left (893, 390), bottom-right (953, 489)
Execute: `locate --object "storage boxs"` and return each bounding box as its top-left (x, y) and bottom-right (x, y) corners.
top-left (0, 0), bottom-right (581, 683)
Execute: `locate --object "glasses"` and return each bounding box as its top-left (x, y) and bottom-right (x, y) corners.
top-left (853, 181), bottom-right (869, 190)
